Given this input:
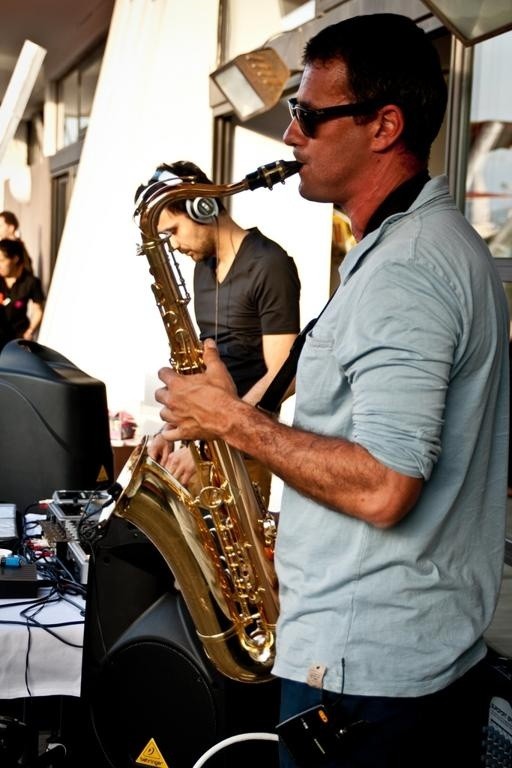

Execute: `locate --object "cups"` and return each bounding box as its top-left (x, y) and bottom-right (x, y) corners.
top-left (289, 97), bottom-right (364, 138)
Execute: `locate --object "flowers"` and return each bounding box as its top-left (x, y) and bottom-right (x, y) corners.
top-left (105, 481), bottom-right (123, 501)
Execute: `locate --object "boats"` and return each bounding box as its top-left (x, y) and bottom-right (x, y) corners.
top-left (0, 338), bottom-right (115, 516)
top-left (76, 509), bottom-right (281, 768)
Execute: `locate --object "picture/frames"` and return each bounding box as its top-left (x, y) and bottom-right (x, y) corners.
top-left (0, 503), bottom-right (103, 768)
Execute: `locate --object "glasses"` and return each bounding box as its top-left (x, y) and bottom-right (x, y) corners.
top-left (208, 47), bottom-right (292, 124)
top-left (9, 164), bottom-right (32, 204)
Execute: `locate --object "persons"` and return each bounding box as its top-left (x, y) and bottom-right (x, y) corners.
top-left (0, 237), bottom-right (45, 353)
top-left (134, 160), bottom-right (301, 510)
top-left (154, 11), bottom-right (512, 766)
top-left (0, 211), bottom-right (34, 274)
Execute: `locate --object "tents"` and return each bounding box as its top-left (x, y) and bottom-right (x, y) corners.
top-left (152, 170), bottom-right (220, 225)
